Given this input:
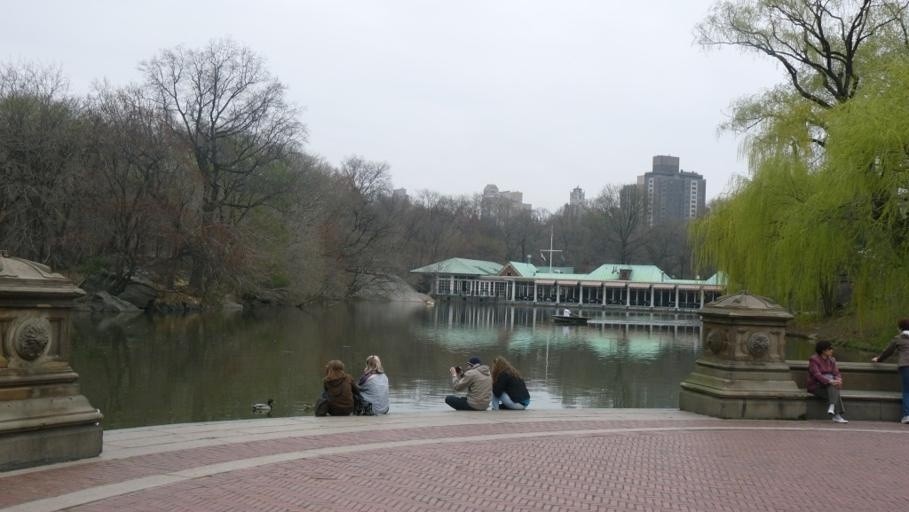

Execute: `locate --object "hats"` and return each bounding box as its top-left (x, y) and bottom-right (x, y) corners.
top-left (466, 356), bottom-right (482, 367)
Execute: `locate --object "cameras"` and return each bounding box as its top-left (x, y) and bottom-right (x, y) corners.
top-left (454, 366), bottom-right (460, 373)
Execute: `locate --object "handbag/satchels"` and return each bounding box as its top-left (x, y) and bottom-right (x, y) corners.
top-left (355, 398), bottom-right (374, 417)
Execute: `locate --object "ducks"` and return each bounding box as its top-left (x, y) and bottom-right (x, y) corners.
top-left (252, 399), bottom-right (276, 411)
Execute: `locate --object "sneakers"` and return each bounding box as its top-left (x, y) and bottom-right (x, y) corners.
top-left (827, 407), bottom-right (848, 424)
top-left (901, 416), bottom-right (909, 424)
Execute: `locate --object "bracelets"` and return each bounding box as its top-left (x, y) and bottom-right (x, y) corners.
top-left (451, 375), bottom-right (456, 377)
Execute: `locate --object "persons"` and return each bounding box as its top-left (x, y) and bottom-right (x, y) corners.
top-left (805, 339), bottom-right (849, 424)
top-left (491, 354), bottom-right (530, 411)
top-left (357, 355), bottom-right (390, 416)
top-left (444, 356), bottom-right (493, 412)
top-left (313, 359), bottom-right (360, 416)
top-left (553, 303), bottom-right (578, 317)
top-left (870, 316), bottom-right (909, 423)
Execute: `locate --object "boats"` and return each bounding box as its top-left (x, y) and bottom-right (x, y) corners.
top-left (554, 314), bottom-right (591, 327)
